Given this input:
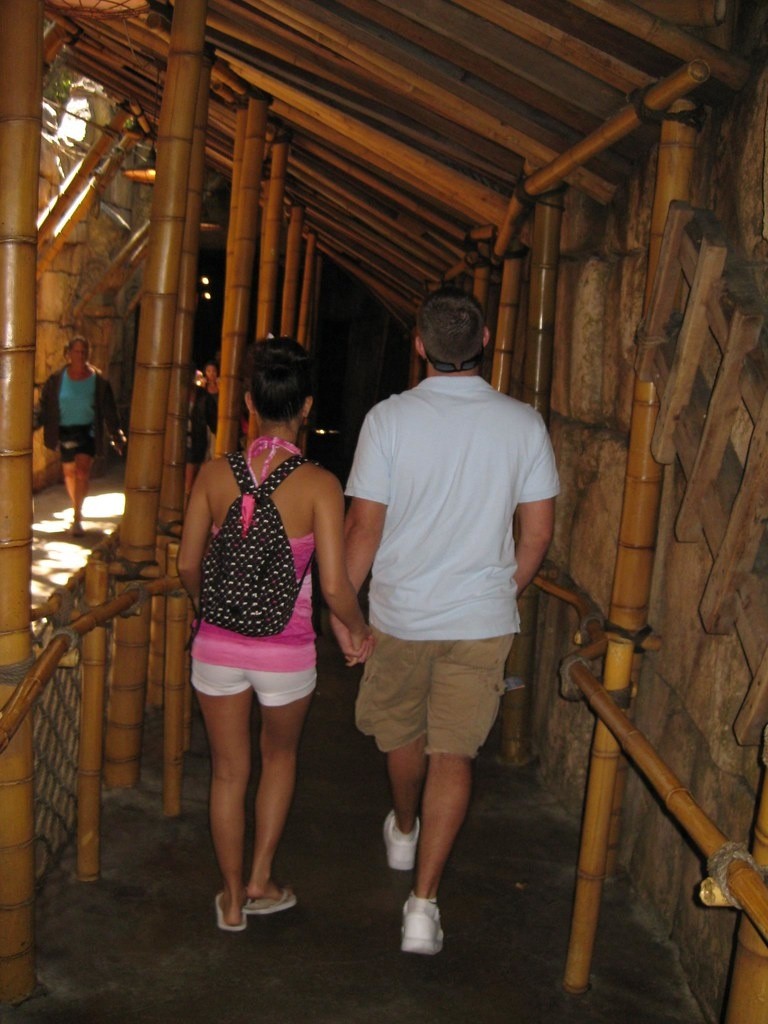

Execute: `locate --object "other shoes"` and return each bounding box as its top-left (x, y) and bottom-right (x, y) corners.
top-left (69, 522), bottom-right (84, 537)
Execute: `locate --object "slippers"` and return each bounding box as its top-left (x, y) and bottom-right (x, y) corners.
top-left (214, 892), bottom-right (248, 932)
top-left (242, 886), bottom-right (296, 914)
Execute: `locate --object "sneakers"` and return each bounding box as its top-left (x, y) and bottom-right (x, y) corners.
top-left (384, 808), bottom-right (419, 871)
top-left (400, 900), bottom-right (443, 955)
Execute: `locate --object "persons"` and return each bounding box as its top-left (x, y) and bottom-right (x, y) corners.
top-left (31, 336), bottom-right (126, 540)
top-left (176, 336), bottom-right (374, 934)
top-left (187, 349), bottom-right (250, 512)
top-left (329, 287), bottom-right (561, 958)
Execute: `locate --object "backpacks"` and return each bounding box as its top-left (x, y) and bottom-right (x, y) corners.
top-left (200, 451), bottom-right (309, 636)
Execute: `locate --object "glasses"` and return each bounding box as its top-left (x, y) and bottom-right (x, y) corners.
top-left (424, 344), bottom-right (487, 372)
